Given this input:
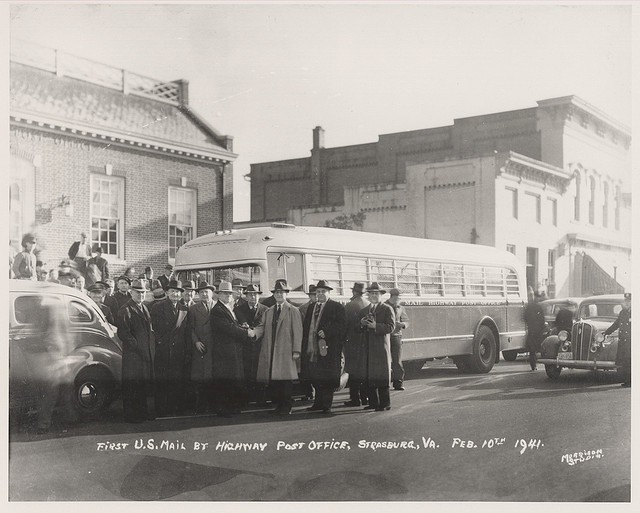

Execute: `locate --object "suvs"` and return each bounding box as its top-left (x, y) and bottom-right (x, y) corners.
top-left (536, 292), bottom-right (624, 379)
top-left (502, 298), bottom-right (597, 361)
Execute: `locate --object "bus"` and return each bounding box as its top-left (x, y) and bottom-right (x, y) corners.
top-left (173, 222), bottom-right (528, 399)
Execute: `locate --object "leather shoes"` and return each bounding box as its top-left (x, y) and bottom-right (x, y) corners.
top-left (322, 406), bottom-right (331, 415)
top-left (270, 407), bottom-right (281, 414)
top-left (307, 403), bottom-right (323, 410)
top-left (395, 385), bottom-right (405, 390)
top-left (364, 404), bottom-right (378, 408)
top-left (374, 404), bottom-right (390, 410)
top-left (361, 397), bottom-right (367, 404)
top-left (278, 408), bottom-right (292, 418)
top-left (346, 400), bottom-right (360, 406)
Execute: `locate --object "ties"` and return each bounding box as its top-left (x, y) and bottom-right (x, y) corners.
top-left (206, 302), bottom-right (210, 312)
top-left (252, 305), bottom-right (256, 316)
top-left (277, 304), bottom-right (281, 314)
top-left (370, 304), bottom-right (375, 312)
top-left (172, 303), bottom-right (177, 313)
top-left (314, 303), bottom-right (321, 327)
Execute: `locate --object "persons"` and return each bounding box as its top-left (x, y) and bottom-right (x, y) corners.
top-left (297, 283), bottom-right (317, 326)
top-left (157, 264), bottom-right (174, 292)
top-left (182, 278), bottom-right (197, 306)
top-left (186, 280), bottom-right (217, 416)
top-left (124, 267), bottom-right (136, 286)
top-left (146, 287), bottom-right (167, 313)
top-left (260, 278), bottom-right (303, 307)
top-left (355, 281), bottom-right (395, 411)
top-left (116, 279), bottom-right (156, 423)
top-left (298, 280), bottom-right (346, 416)
top-left (113, 275), bottom-right (131, 307)
top-left (9, 231), bottom-right (117, 324)
top-left (555, 304), bottom-right (575, 336)
top-left (211, 282), bottom-right (256, 417)
top-left (144, 266), bottom-right (154, 279)
top-left (149, 280), bottom-right (191, 417)
top-left (344, 282), bottom-right (371, 407)
top-left (602, 293), bottom-right (631, 388)
top-left (253, 282), bottom-right (304, 419)
top-left (232, 278), bottom-right (247, 308)
top-left (384, 288), bottom-right (410, 391)
top-left (234, 284), bottom-right (269, 328)
top-left (526, 291), bottom-right (545, 371)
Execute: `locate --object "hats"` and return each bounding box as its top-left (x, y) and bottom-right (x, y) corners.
top-left (232, 278), bottom-right (248, 287)
top-left (270, 279), bottom-right (289, 292)
top-left (95, 281), bottom-right (110, 289)
top-left (87, 283), bottom-right (103, 291)
top-left (306, 284), bottom-right (316, 294)
top-left (350, 282), bottom-right (366, 293)
top-left (313, 279), bottom-right (334, 290)
top-left (166, 279), bottom-right (184, 292)
top-left (183, 280), bottom-right (199, 290)
top-left (153, 287), bottom-right (167, 300)
top-left (58, 260), bottom-right (71, 267)
top-left (214, 281), bottom-right (236, 292)
top-left (118, 275), bottom-right (131, 284)
top-left (367, 281), bottom-right (386, 294)
top-left (164, 264), bottom-right (173, 271)
top-left (36, 260), bottom-right (47, 266)
top-left (196, 280), bottom-right (216, 291)
top-left (389, 288), bottom-right (402, 294)
top-left (131, 278), bottom-right (151, 291)
top-left (624, 292), bottom-right (631, 300)
top-left (243, 283), bottom-right (263, 293)
top-left (25, 236), bottom-right (36, 243)
top-left (96, 247), bottom-right (103, 253)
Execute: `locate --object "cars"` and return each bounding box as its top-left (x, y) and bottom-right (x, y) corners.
top-left (8, 278), bottom-right (123, 414)
